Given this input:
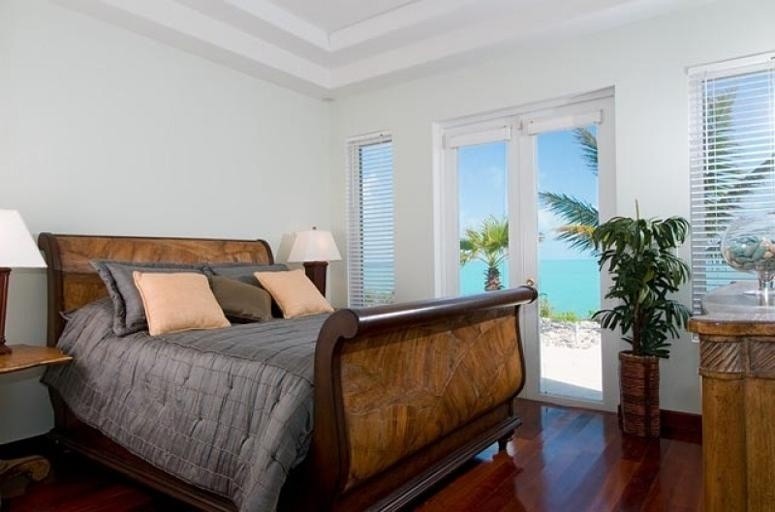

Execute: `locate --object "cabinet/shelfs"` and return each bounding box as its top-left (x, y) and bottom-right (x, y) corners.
top-left (687, 281), bottom-right (775, 512)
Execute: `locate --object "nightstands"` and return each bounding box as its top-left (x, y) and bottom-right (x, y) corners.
top-left (0, 343), bottom-right (73, 484)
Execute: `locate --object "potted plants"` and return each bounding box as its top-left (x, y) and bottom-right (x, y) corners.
top-left (594, 201), bottom-right (695, 446)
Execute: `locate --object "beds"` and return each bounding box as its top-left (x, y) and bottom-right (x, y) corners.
top-left (39, 231), bottom-right (538, 511)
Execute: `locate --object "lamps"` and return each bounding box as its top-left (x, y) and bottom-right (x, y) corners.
top-left (0, 208), bottom-right (48, 356)
top-left (287, 227), bottom-right (343, 299)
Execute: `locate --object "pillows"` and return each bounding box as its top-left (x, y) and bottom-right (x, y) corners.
top-left (133, 270), bottom-right (231, 337)
top-left (210, 263), bottom-right (290, 318)
top-left (208, 275), bottom-right (272, 323)
top-left (254, 268), bottom-right (335, 320)
top-left (90, 258), bottom-right (214, 338)
